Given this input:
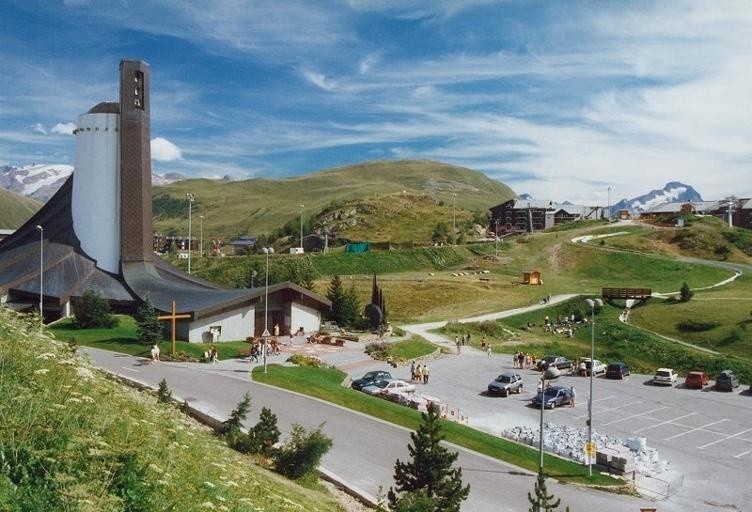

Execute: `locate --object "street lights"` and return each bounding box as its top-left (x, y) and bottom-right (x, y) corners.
top-left (607, 185), bottom-right (612, 223)
top-left (452, 192), bottom-right (457, 233)
top-left (186, 192), bottom-right (195, 274)
top-left (36, 225), bottom-right (44, 333)
top-left (538, 374), bottom-right (547, 467)
top-left (299, 204), bottom-right (304, 248)
top-left (581, 298), bottom-right (603, 476)
top-left (199, 215), bottom-right (204, 257)
top-left (262, 247), bottom-right (274, 337)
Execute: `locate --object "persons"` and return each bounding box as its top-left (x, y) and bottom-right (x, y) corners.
top-left (544, 312), bottom-right (575, 338)
top-left (273, 322), bottom-right (280, 336)
top-left (540, 358), bottom-right (547, 375)
top-left (566, 360), bottom-right (589, 376)
top-left (513, 351), bottom-right (536, 368)
top-left (210, 346), bottom-right (219, 364)
top-left (150, 344), bottom-right (161, 365)
top-left (481, 336), bottom-right (492, 359)
top-left (409, 360), bottom-right (430, 384)
top-left (456, 329), bottom-right (472, 346)
top-left (296, 325), bottom-right (323, 344)
top-left (537, 381), bottom-right (553, 394)
top-left (568, 386), bottom-right (576, 407)
top-left (247, 339), bottom-right (280, 364)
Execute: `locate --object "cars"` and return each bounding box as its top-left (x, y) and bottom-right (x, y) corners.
top-left (537, 356), bottom-right (630, 379)
top-left (653, 367), bottom-right (739, 391)
top-left (488, 373), bottom-right (523, 397)
top-left (532, 386), bottom-right (571, 409)
top-left (351, 371), bottom-right (415, 395)
